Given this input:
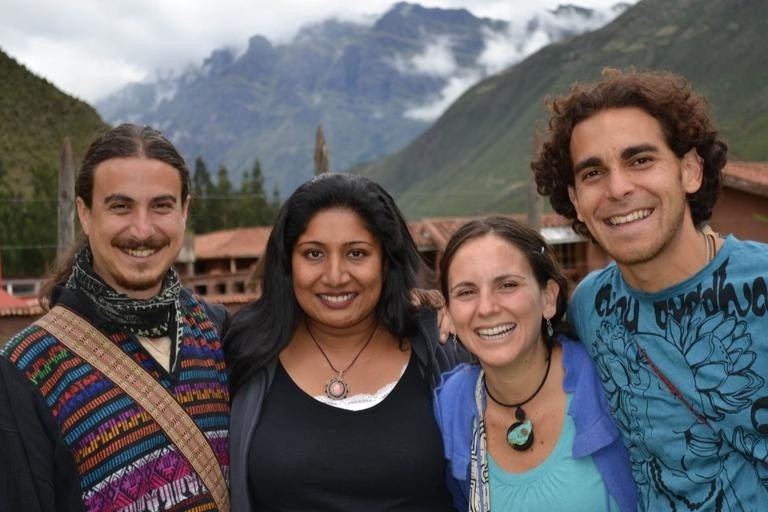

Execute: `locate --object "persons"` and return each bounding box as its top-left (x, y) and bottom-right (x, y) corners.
top-left (427, 214), bottom-right (645, 511)
top-left (226, 172), bottom-right (465, 509)
top-left (528, 66), bottom-right (768, 511)
top-left (0, 122), bottom-right (457, 512)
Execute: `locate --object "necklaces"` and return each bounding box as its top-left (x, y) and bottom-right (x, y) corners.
top-left (482, 343), bottom-right (558, 453)
top-left (706, 233), bottom-right (718, 258)
top-left (700, 229), bottom-right (711, 268)
top-left (298, 317), bottom-right (381, 403)
